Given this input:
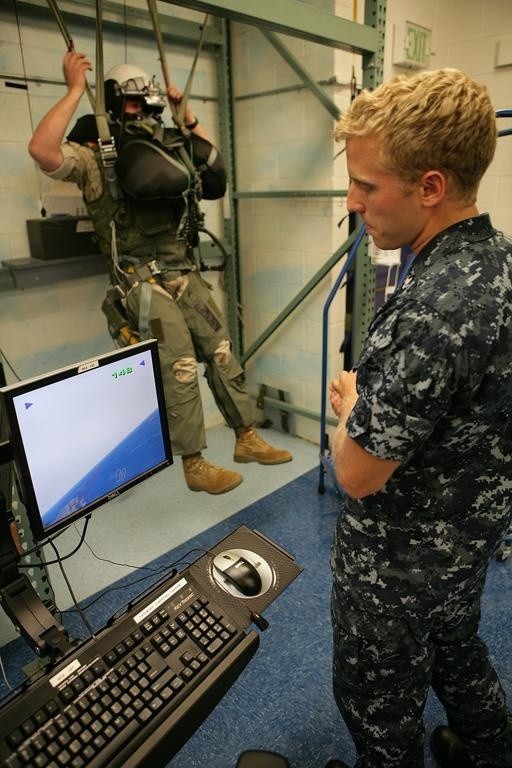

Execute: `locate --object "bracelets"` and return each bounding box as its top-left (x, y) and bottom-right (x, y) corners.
top-left (186, 115), bottom-right (199, 130)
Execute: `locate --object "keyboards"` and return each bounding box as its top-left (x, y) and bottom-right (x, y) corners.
top-left (0, 570), bottom-right (260, 768)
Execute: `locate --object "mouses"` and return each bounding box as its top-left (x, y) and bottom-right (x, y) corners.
top-left (213, 550), bottom-right (262, 596)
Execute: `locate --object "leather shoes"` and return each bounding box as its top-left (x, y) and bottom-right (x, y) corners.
top-left (430, 724), bottom-right (475, 768)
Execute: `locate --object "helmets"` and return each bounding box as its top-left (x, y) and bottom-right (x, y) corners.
top-left (104, 64), bottom-right (168, 109)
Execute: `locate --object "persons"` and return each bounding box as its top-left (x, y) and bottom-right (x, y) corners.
top-left (27, 48), bottom-right (295, 495)
top-left (326, 66), bottom-right (510, 768)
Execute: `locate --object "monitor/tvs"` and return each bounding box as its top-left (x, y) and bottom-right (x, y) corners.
top-left (0, 339), bottom-right (174, 541)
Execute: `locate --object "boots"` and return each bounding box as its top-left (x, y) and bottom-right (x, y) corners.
top-left (182, 451), bottom-right (244, 494)
top-left (233, 425), bottom-right (293, 464)
